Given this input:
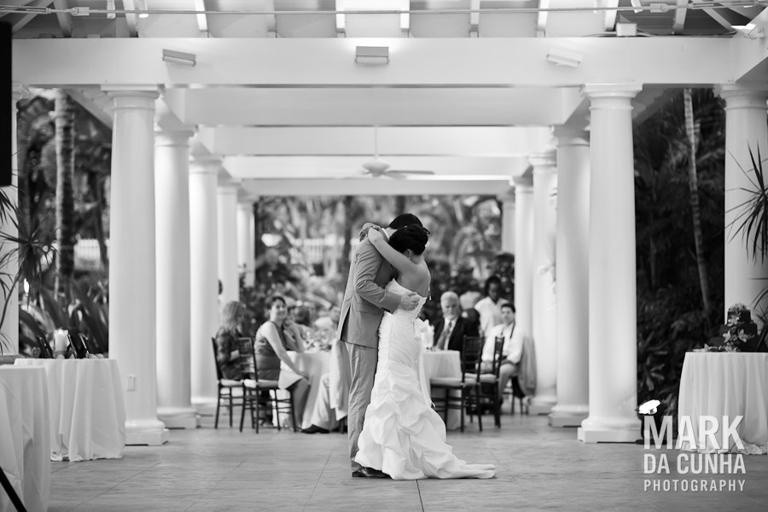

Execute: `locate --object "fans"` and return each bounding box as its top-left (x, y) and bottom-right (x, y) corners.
top-left (357, 128), bottom-right (435, 182)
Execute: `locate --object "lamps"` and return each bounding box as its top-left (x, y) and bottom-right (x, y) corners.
top-left (354, 45), bottom-right (390, 66)
top-left (160, 48), bottom-right (196, 67)
top-left (546, 48), bottom-right (581, 69)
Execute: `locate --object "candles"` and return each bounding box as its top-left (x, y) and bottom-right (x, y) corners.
top-left (54, 328), bottom-right (65, 352)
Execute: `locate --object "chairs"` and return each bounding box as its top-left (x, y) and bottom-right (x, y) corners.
top-left (430, 335), bottom-right (483, 433)
top-left (505, 336), bottom-right (532, 415)
top-left (237, 337), bottom-right (297, 434)
top-left (465, 336), bottom-right (504, 428)
top-left (211, 337), bottom-right (255, 428)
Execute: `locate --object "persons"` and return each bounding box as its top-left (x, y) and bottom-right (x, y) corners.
top-left (355, 222), bottom-right (495, 481)
top-left (434, 276), bottom-right (523, 407)
top-left (214, 295), bottom-right (340, 434)
top-left (339, 213), bottom-right (422, 480)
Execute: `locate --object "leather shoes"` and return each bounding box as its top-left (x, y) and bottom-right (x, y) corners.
top-left (352, 467), bottom-right (387, 478)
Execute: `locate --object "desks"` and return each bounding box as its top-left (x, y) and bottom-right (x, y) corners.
top-left (0, 365), bottom-right (51, 512)
top-left (674, 352), bottom-right (768, 454)
top-left (14, 358), bottom-right (127, 462)
top-left (276, 350), bottom-right (463, 431)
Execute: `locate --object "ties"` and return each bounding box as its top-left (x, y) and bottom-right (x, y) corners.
top-left (439, 323), bottom-right (452, 350)
